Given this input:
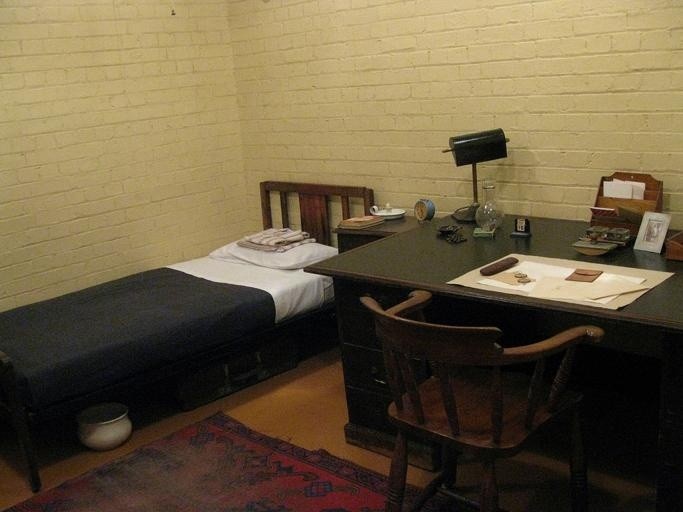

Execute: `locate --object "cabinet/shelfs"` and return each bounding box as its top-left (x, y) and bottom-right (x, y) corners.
top-left (332, 216), bottom-right (440, 255)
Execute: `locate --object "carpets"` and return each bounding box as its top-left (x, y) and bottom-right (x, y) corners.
top-left (0, 411), bottom-right (480, 512)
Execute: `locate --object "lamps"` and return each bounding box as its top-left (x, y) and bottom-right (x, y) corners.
top-left (442, 127), bottom-right (510, 223)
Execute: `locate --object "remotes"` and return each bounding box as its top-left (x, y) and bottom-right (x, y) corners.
top-left (480, 256), bottom-right (519, 275)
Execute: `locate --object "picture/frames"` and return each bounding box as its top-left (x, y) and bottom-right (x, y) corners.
top-left (634, 211), bottom-right (672, 254)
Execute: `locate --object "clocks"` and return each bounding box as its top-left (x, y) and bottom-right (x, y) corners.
top-left (414, 198), bottom-right (435, 223)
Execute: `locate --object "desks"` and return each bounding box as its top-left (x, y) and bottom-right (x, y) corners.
top-left (303, 210), bottom-right (683, 512)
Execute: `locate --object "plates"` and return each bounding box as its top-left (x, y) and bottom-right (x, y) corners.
top-left (374, 209), bottom-right (406, 220)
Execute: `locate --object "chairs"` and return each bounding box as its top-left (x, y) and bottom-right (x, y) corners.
top-left (359, 290), bottom-right (606, 512)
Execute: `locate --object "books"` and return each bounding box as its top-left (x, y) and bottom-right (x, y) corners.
top-left (590, 206), bottom-right (617, 219)
top-left (340, 217), bottom-right (384, 230)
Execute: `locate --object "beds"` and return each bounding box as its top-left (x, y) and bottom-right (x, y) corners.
top-left (0, 180), bottom-right (373, 493)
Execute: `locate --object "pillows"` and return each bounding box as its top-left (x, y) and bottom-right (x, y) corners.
top-left (209, 234), bottom-right (338, 270)
